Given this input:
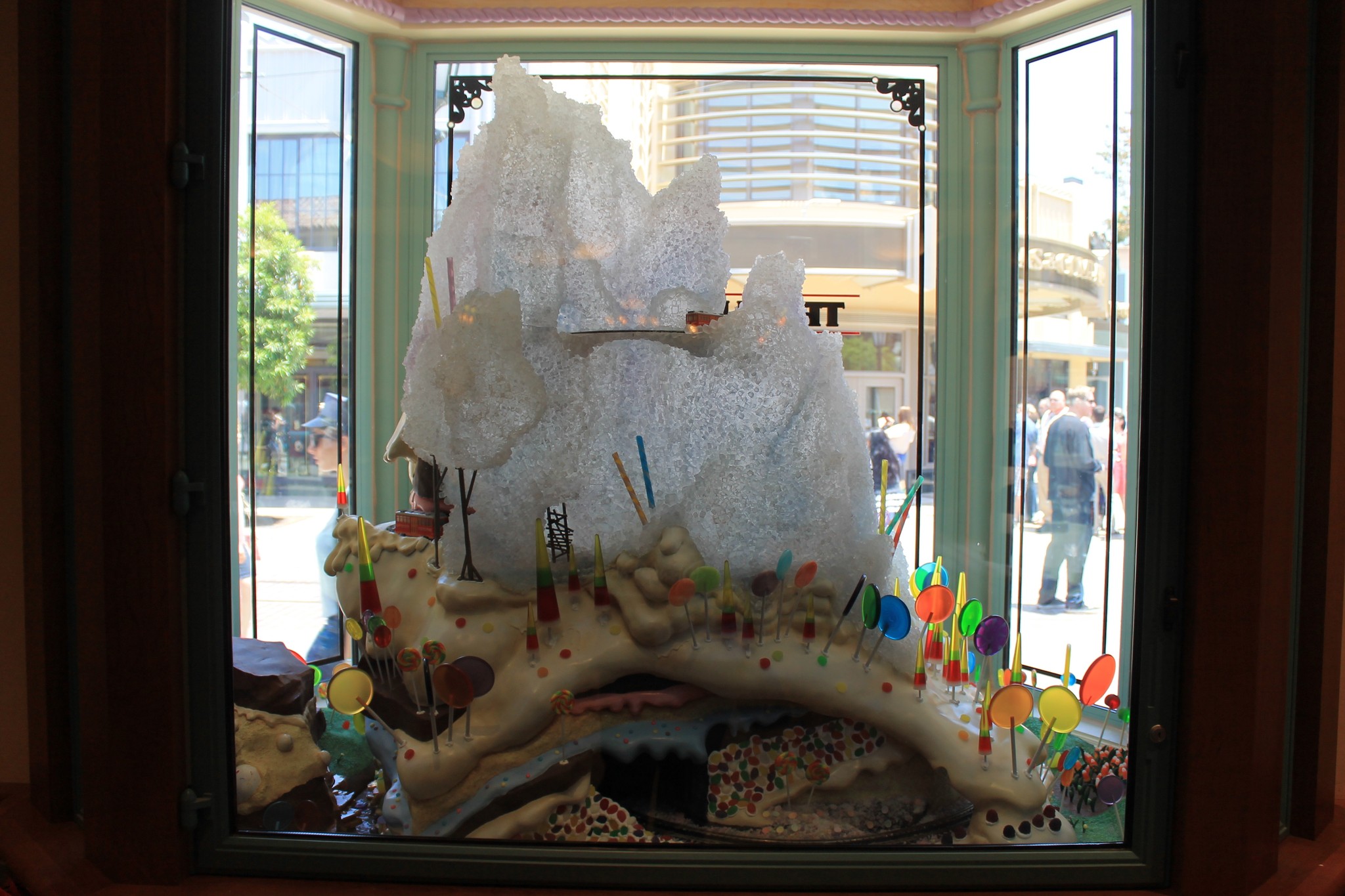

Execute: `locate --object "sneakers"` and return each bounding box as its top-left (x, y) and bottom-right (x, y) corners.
top-left (1036, 598), bottom-right (1066, 609)
top-left (1063, 601), bottom-right (1100, 614)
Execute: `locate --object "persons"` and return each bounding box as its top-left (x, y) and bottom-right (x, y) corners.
top-left (879, 385), bottom-right (1127, 543)
top-left (238, 404), bottom-right (288, 638)
top-left (299, 390), bottom-right (351, 684)
top-left (866, 430), bottom-right (906, 541)
top-left (1036, 384), bottom-right (1107, 612)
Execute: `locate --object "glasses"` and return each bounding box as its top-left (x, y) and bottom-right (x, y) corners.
top-left (1080, 397), bottom-right (1096, 404)
top-left (307, 430), bottom-right (339, 447)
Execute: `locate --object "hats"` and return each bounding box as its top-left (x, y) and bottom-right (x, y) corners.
top-left (301, 391), bottom-right (351, 434)
